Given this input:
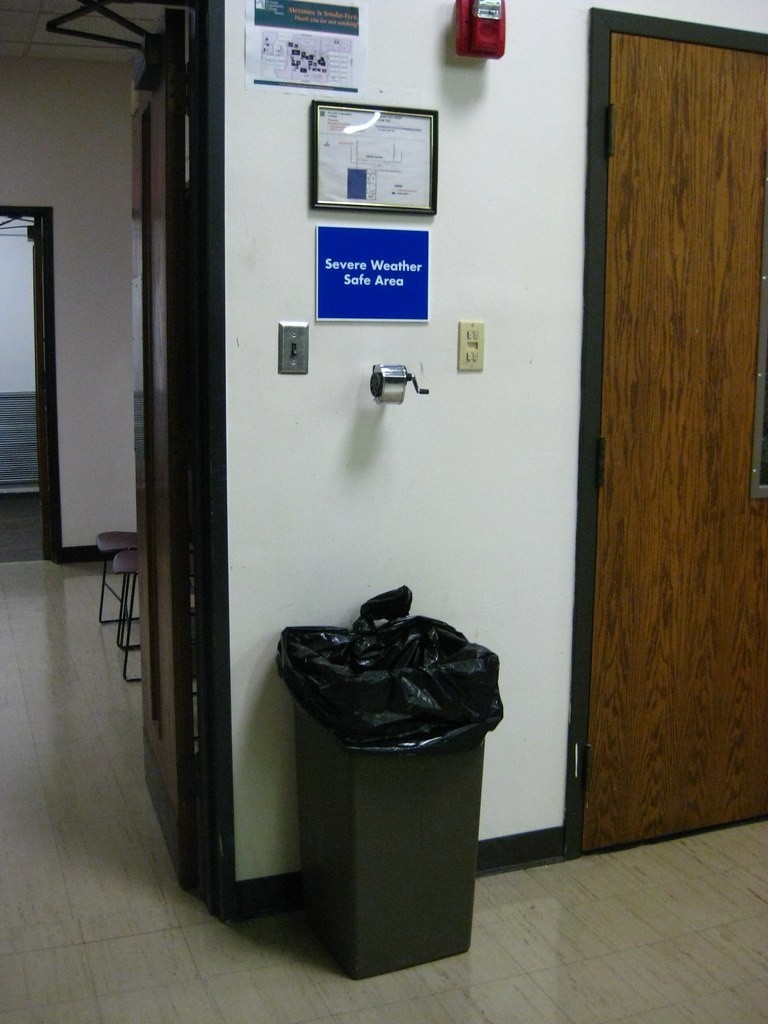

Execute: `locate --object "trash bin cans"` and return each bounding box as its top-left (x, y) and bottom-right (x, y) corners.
top-left (279, 615), bottom-right (504, 981)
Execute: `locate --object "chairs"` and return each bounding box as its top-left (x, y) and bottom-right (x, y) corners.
top-left (95, 531), bottom-right (140, 683)
